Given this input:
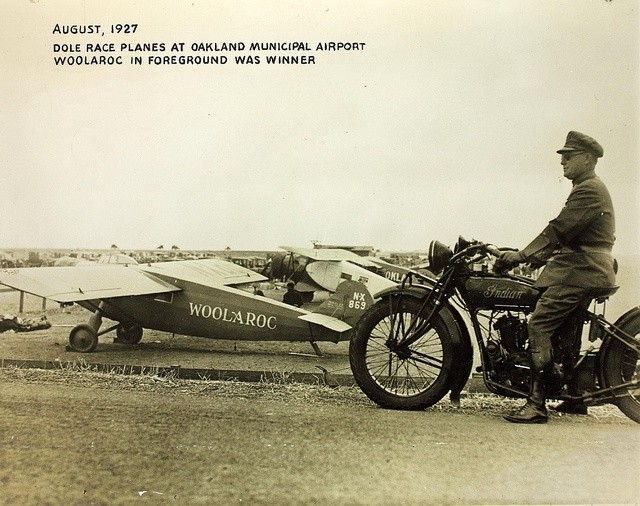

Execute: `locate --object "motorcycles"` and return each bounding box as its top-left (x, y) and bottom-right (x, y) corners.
top-left (348, 232), bottom-right (639, 425)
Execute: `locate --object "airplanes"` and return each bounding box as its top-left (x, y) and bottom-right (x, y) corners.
top-left (271, 246), bottom-right (447, 303)
top-left (0, 252), bottom-right (356, 357)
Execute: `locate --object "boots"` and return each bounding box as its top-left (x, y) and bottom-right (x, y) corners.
top-left (548, 387), bottom-right (587, 415)
top-left (501, 367), bottom-right (548, 424)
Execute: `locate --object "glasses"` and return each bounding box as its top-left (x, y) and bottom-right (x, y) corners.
top-left (564, 152), bottom-right (584, 160)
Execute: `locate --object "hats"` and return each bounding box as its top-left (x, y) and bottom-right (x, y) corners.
top-left (557, 131), bottom-right (603, 158)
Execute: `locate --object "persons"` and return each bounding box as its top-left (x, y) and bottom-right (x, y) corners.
top-left (282, 282), bottom-right (303, 308)
top-left (492, 130), bottom-right (619, 425)
